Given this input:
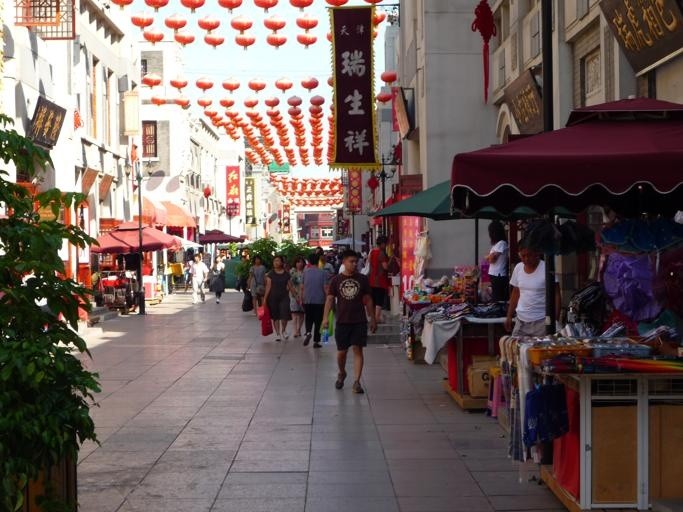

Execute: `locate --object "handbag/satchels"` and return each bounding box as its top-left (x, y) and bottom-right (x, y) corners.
top-left (261, 307), bottom-right (272, 336)
top-left (360, 248), bottom-right (373, 276)
top-left (254, 285), bottom-right (264, 296)
top-left (561, 282), bottom-right (605, 333)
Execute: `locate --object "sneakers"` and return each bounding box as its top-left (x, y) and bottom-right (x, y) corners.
top-left (275, 335), bottom-right (280, 340)
top-left (282, 332), bottom-right (288, 338)
top-left (351, 384), bottom-right (362, 393)
top-left (191, 300), bottom-right (221, 304)
top-left (335, 372), bottom-right (346, 389)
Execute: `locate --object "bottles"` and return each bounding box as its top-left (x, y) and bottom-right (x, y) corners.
top-left (411, 283), bottom-right (423, 302)
top-left (322, 324), bottom-right (329, 345)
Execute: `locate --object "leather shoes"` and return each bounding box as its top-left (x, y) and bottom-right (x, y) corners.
top-left (303, 334), bottom-right (311, 345)
top-left (313, 343), bottom-right (321, 348)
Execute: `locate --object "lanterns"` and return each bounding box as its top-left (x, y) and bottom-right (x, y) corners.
top-left (151, 95), bottom-right (325, 109)
top-left (270, 174), bottom-right (345, 205)
top-left (144, 75), bottom-right (333, 93)
top-left (377, 72), bottom-right (397, 102)
top-left (374, 11), bottom-right (386, 39)
top-left (205, 105), bottom-right (334, 166)
top-left (113, 0), bottom-right (381, 13)
top-left (368, 176), bottom-right (378, 188)
top-left (130, 11), bottom-right (333, 49)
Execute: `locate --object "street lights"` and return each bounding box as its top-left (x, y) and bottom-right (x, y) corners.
top-left (130, 159), bottom-right (149, 315)
top-left (370, 151), bottom-right (398, 264)
top-left (344, 198), bottom-right (359, 254)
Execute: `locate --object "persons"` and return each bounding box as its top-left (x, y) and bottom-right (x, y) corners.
top-left (182, 233), bottom-right (397, 394)
top-left (503, 236), bottom-right (562, 336)
top-left (486, 221), bottom-right (510, 301)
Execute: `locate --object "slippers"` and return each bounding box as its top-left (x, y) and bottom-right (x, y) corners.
top-left (374, 319), bottom-right (386, 324)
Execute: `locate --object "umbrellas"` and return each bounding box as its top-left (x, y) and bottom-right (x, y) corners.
top-left (372, 179), bottom-right (577, 266)
top-left (91, 221), bottom-right (177, 254)
top-left (173, 235), bottom-right (204, 251)
